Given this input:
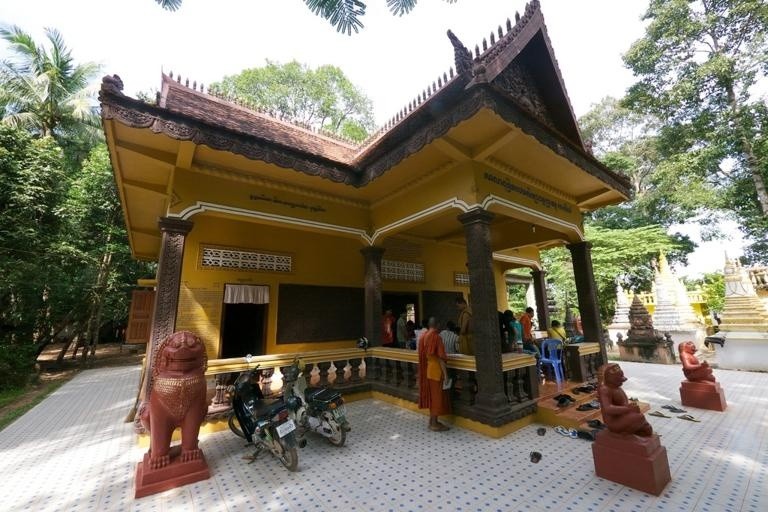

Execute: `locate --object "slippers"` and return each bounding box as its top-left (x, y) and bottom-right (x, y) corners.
top-left (529, 451), bottom-right (542, 463)
top-left (648, 405), bottom-right (701, 422)
top-left (554, 381), bottom-right (607, 442)
top-left (537, 427), bottom-right (546, 436)
top-left (431, 425), bottom-right (449, 431)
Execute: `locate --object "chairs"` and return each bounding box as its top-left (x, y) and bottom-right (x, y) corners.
top-left (537, 339), bottom-right (565, 384)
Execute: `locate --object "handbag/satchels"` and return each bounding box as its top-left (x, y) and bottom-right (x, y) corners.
top-left (426, 355), bottom-right (442, 382)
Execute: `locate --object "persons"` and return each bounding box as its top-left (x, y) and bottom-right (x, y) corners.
top-left (678, 339), bottom-right (715, 384)
top-left (382, 296), bottom-right (568, 432)
top-left (597, 361), bottom-right (654, 438)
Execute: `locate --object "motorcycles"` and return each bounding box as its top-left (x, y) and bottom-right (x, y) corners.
top-left (273, 355), bottom-right (351, 446)
top-left (226, 353), bottom-right (307, 471)
